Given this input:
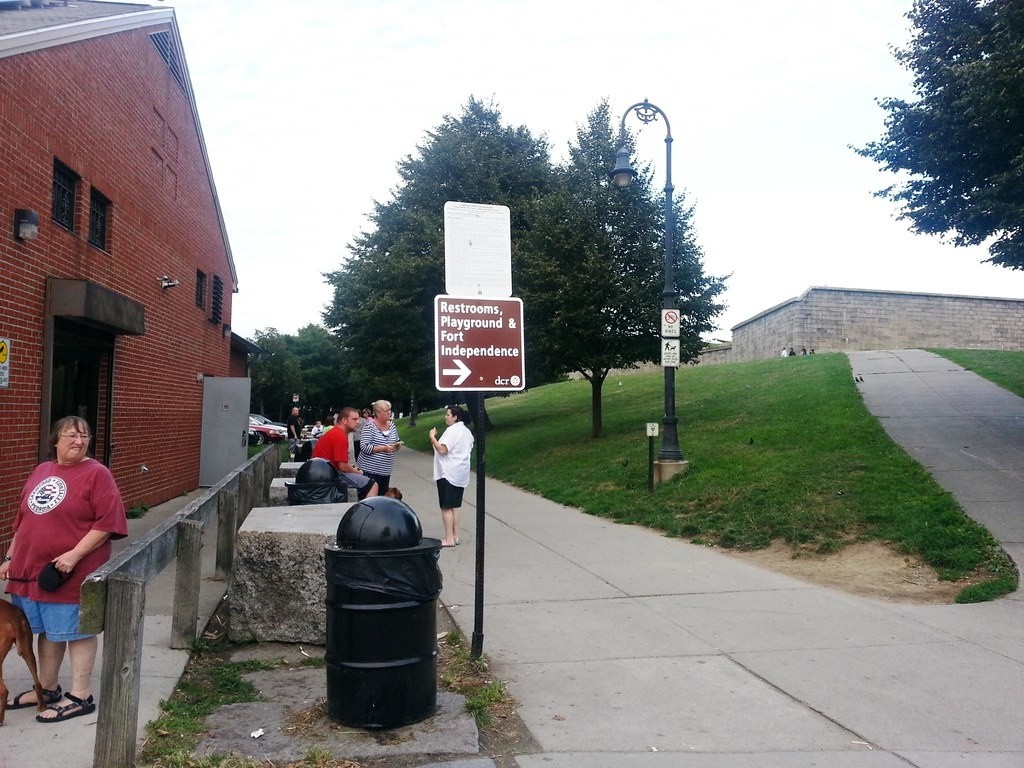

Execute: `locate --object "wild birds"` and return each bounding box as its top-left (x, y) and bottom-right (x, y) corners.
top-left (855, 375), bottom-right (865, 383)
top-left (748, 437), bottom-right (754, 445)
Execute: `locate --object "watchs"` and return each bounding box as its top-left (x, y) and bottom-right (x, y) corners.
top-left (4, 556), bottom-right (11, 561)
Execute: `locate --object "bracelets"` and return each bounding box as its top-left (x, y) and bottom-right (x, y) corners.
top-left (431, 438), bottom-right (436, 444)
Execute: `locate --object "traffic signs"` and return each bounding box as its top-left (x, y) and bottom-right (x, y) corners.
top-left (432, 294), bottom-right (525, 392)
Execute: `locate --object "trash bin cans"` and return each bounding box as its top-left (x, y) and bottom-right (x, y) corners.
top-left (323, 496), bottom-right (442, 730)
top-left (284, 458), bottom-right (349, 506)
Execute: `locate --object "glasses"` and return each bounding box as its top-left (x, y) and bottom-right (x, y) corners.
top-left (61, 433), bottom-right (92, 441)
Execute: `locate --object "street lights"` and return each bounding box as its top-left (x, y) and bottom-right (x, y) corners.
top-left (610, 96), bottom-right (685, 465)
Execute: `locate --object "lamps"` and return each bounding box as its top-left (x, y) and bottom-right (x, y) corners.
top-left (223, 324), bottom-right (231, 338)
top-left (14, 209), bottom-right (39, 242)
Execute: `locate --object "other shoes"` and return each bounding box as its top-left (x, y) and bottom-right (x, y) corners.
top-left (441, 537), bottom-right (460, 547)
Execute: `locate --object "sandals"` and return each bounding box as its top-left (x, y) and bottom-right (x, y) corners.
top-left (36, 692), bottom-right (95, 722)
top-left (5, 685), bottom-right (62, 710)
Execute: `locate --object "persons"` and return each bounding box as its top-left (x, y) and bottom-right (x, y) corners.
top-left (0, 416), bottom-right (128, 722)
top-left (312, 400), bottom-right (403, 502)
top-left (781, 347), bottom-right (815, 357)
top-left (429, 405), bottom-right (474, 548)
top-left (288, 407), bottom-right (302, 462)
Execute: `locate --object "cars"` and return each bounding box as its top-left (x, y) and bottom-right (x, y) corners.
top-left (249, 414), bottom-right (289, 438)
top-left (304, 425), bottom-right (314, 433)
top-left (248, 428), bottom-right (259, 444)
top-left (249, 416), bottom-right (286, 446)
